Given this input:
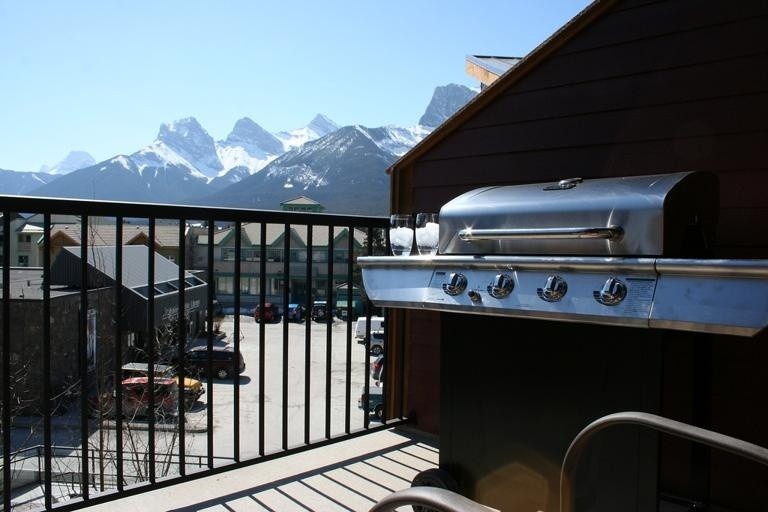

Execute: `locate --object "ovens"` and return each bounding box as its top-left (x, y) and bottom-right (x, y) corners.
top-left (424, 169), bottom-right (720, 329)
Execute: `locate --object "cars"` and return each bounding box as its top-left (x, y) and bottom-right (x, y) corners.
top-left (288, 303), bottom-right (306, 322)
top-left (358, 330), bottom-right (385, 421)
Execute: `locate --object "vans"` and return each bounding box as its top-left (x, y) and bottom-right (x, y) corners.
top-left (354, 316), bottom-right (384, 344)
top-left (184, 345), bottom-right (247, 380)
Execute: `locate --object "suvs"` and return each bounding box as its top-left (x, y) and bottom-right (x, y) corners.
top-left (120, 362), bottom-right (206, 412)
top-left (253, 302), bottom-right (279, 323)
top-left (311, 301), bottom-right (332, 322)
top-left (89, 376), bottom-right (180, 425)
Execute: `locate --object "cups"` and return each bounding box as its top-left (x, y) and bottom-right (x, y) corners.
top-left (389, 212), bottom-right (441, 260)
